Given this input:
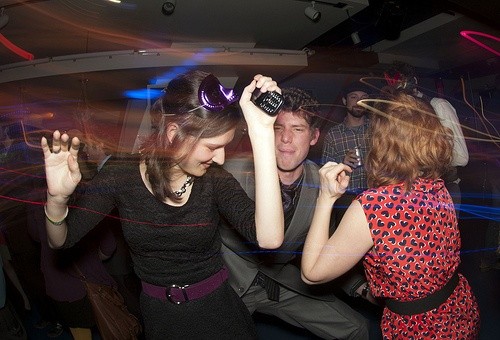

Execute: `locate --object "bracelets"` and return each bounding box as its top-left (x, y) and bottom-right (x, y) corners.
top-left (43, 204), bottom-right (68, 225)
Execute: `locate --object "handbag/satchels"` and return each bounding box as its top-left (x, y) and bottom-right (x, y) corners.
top-left (82, 278), bottom-right (142, 340)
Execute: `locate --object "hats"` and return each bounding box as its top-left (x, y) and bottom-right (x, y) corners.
top-left (343, 81), bottom-right (370, 96)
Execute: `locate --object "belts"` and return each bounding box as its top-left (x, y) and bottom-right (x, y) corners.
top-left (141, 263), bottom-right (231, 303)
top-left (383, 270), bottom-right (460, 316)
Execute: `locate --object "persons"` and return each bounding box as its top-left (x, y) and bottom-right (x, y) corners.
top-left (0, 61), bottom-right (480, 340)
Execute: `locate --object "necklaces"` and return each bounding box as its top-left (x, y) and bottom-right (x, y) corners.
top-left (174, 174), bottom-right (194, 195)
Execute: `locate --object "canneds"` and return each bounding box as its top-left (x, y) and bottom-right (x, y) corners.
top-left (353, 146), bottom-right (363, 167)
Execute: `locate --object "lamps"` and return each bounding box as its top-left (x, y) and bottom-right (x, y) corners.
top-left (162, 0), bottom-right (176, 15)
top-left (304, 1), bottom-right (320, 22)
top-left (351, 32), bottom-right (361, 45)
